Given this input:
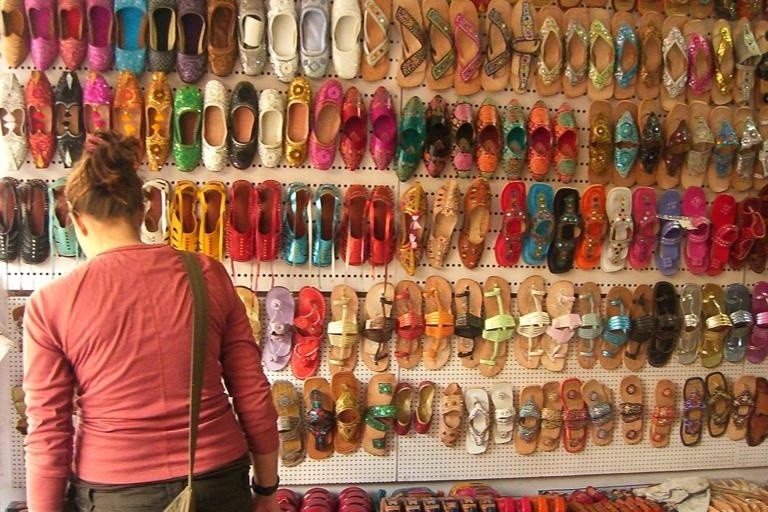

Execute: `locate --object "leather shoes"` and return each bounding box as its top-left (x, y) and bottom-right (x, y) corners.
top-left (367, 87), bottom-right (581, 182)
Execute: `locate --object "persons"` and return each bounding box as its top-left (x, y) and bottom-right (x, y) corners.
top-left (21, 131), bottom-right (280, 512)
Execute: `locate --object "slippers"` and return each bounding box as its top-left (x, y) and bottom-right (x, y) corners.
top-left (233, 275), bottom-right (768, 467)
top-left (362, 0), bottom-right (767, 105)
top-left (588, 99), bottom-right (767, 193)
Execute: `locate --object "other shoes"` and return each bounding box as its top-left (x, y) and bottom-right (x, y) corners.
top-left (21, 179), bottom-right (50, 263)
top-left (2, 0), bottom-right (362, 173)
top-left (47, 176), bottom-right (81, 257)
top-left (0, 176), bottom-right (22, 263)
top-left (142, 178), bottom-right (763, 272)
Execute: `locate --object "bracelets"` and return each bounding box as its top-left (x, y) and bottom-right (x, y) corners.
top-left (252, 475), bottom-right (279, 496)
